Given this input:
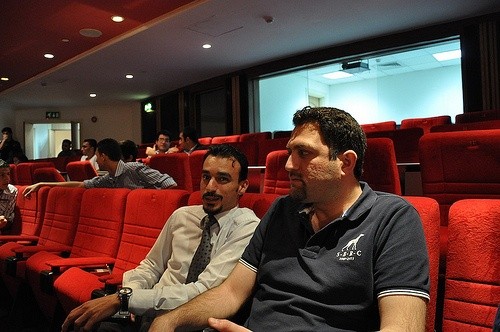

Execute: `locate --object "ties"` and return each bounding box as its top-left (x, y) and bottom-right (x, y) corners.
top-left (186, 215), bottom-right (217, 284)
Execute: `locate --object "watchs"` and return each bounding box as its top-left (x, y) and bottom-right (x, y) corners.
top-left (116, 287), bottom-right (133, 312)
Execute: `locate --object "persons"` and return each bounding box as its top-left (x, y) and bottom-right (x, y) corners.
top-left (61, 144), bottom-right (261, 331)
top-left (0, 127), bottom-right (207, 198)
top-left (0, 158), bottom-right (19, 234)
top-left (147, 106), bottom-right (430, 332)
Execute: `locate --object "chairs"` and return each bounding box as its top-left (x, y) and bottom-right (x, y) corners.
top-left (0, 108), bottom-right (500, 332)
